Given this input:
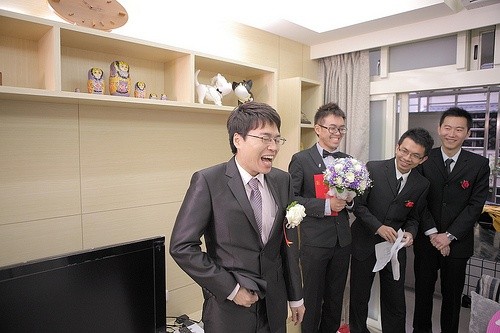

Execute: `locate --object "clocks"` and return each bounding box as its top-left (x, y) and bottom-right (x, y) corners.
top-left (48, 0), bottom-right (129, 30)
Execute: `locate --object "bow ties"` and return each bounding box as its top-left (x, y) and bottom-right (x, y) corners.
top-left (323, 149), bottom-right (341, 159)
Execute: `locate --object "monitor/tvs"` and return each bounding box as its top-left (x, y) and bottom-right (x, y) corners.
top-left (0, 236), bottom-right (167, 333)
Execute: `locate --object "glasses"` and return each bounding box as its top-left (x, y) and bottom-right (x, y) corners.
top-left (318, 124), bottom-right (348, 134)
top-left (239, 133), bottom-right (286, 145)
top-left (399, 147), bottom-right (426, 161)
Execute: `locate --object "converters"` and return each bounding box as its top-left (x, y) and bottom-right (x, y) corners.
top-left (176, 314), bottom-right (188, 324)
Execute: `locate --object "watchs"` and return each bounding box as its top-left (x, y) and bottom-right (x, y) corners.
top-left (445, 232), bottom-right (454, 241)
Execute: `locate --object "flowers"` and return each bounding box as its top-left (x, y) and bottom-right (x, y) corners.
top-left (405, 200), bottom-right (415, 208)
top-left (461, 178), bottom-right (470, 189)
top-left (322, 156), bottom-right (374, 200)
top-left (285, 200), bottom-right (307, 229)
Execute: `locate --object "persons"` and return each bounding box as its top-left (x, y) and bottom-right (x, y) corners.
top-left (348, 128), bottom-right (433, 333)
top-left (169, 103), bottom-right (305, 333)
top-left (288, 103), bottom-right (355, 333)
top-left (412, 107), bottom-right (491, 333)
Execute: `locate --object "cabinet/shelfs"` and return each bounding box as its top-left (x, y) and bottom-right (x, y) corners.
top-left (0, 8), bottom-right (275, 112)
top-left (278, 76), bottom-right (320, 171)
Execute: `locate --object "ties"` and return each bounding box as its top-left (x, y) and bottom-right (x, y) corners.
top-left (445, 158), bottom-right (454, 176)
top-left (396, 177), bottom-right (403, 194)
top-left (248, 178), bottom-right (262, 237)
